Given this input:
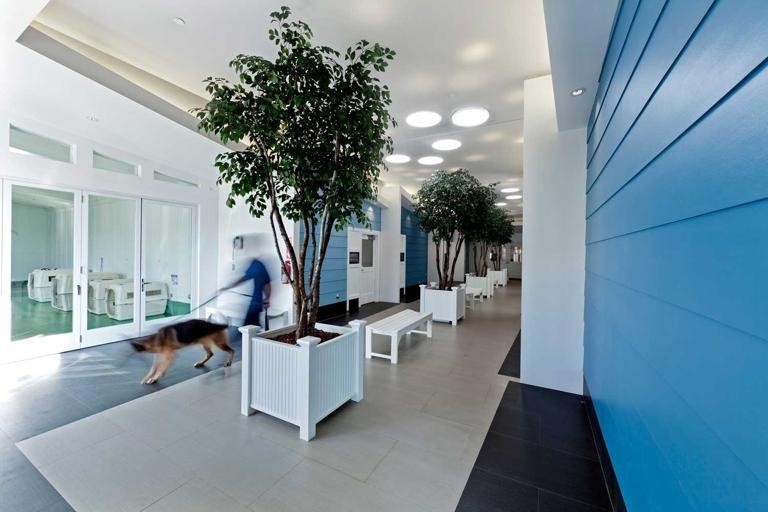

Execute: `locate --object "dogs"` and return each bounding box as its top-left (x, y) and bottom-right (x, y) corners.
top-left (128, 319), bottom-right (235, 386)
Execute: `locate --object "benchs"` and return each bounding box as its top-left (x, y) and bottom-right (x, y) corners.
top-left (365, 309), bottom-right (434, 363)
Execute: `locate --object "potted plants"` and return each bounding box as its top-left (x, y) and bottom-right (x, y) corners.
top-left (410, 170), bottom-right (516, 325)
top-left (190, 4), bottom-right (399, 443)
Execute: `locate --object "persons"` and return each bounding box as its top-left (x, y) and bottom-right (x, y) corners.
top-left (221, 259), bottom-right (274, 336)
